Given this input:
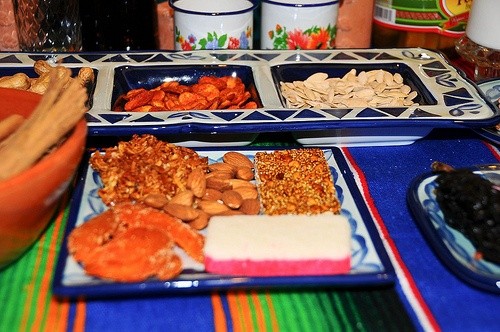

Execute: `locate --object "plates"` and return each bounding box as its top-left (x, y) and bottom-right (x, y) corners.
top-left (407, 165), bottom-right (500, 294)
top-left (470, 78), bottom-right (500, 145)
top-left (52, 147), bottom-right (395, 297)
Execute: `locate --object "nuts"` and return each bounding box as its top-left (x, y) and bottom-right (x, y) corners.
top-left (279, 68), bottom-right (417, 109)
top-left (123, 76), bottom-right (257, 112)
top-left (0, 60), bottom-right (94, 95)
top-left (144, 152), bottom-right (259, 229)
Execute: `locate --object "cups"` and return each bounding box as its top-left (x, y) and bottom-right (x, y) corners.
top-left (455, 0), bottom-right (500, 68)
top-left (260, 0), bottom-right (341, 50)
top-left (11, 0), bottom-right (82, 54)
top-left (169, 0), bottom-right (259, 50)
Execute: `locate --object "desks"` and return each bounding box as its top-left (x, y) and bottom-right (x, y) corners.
top-left (0, 139), bottom-right (500, 332)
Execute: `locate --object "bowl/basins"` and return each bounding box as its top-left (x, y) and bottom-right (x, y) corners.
top-left (0, 88), bottom-right (87, 271)
